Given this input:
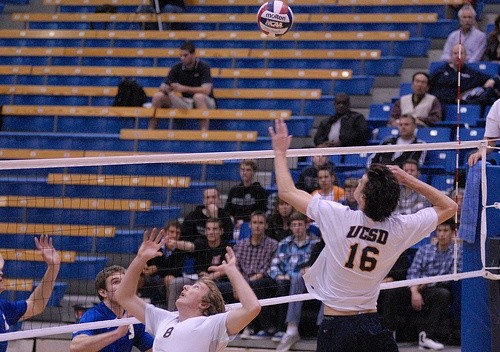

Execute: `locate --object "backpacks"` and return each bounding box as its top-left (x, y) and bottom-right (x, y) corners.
top-left (113, 79), bottom-right (148, 106)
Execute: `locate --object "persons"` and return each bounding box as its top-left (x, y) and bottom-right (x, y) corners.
top-left (268, 118), bottom-right (458, 352)
top-left (387, 72), bottom-right (441, 128)
top-left (450, 0), bottom-right (484, 20)
top-left (139, 0), bottom-right (187, 30)
top-left (138, 156), bottom-right (359, 352)
top-left (430, 44), bottom-right (500, 106)
top-left (440, 6), bottom-right (488, 62)
top-left (69, 265), bottom-right (154, 352)
top-left (0, 234), bottom-right (60, 352)
top-left (148, 43), bottom-right (218, 129)
top-left (314, 93), bottom-right (369, 148)
top-left (468, 99), bottom-right (500, 166)
top-left (485, 15), bottom-right (500, 61)
top-left (395, 159), bottom-right (432, 215)
top-left (377, 251), bottom-right (412, 327)
top-left (407, 218), bottom-right (463, 351)
top-left (372, 113), bottom-right (430, 165)
top-left (116, 228), bottom-right (261, 352)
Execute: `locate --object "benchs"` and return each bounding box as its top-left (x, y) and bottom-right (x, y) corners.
top-left (0, 0), bottom-right (487, 306)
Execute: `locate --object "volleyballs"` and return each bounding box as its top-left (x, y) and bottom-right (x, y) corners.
top-left (256, 0), bottom-right (294, 38)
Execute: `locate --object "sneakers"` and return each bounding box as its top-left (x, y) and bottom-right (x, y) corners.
top-left (276, 332), bottom-right (301, 352)
top-left (240, 327), bottom-right (256, 339)
top-left (272, 332), bottom-right (285, 342)
top-left (251, 330), bottom-right (278, 339)
top-left (418, 331), bottom-right (445, 350)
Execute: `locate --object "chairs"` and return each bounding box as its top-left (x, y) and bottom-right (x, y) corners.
top-left (466, 62), bottom-right (500, 76)
top-left (419, 150), bottom-right (456, 175)
top-left (416, 127), bottom-right (450, 143)
top-left (431, 176), bottom-right (456, 190)
top-left (456, 127), bottom-right (485, 141)
top-left (367, 103), bottom-right (393, 128)
top-left (435, 105), bottom-right (481, 128)
top-left (392, 84), bottom-right (412, 103)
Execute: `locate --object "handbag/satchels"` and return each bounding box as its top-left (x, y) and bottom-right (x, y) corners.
top-left (455, 86), bottom-right (500, 118)
